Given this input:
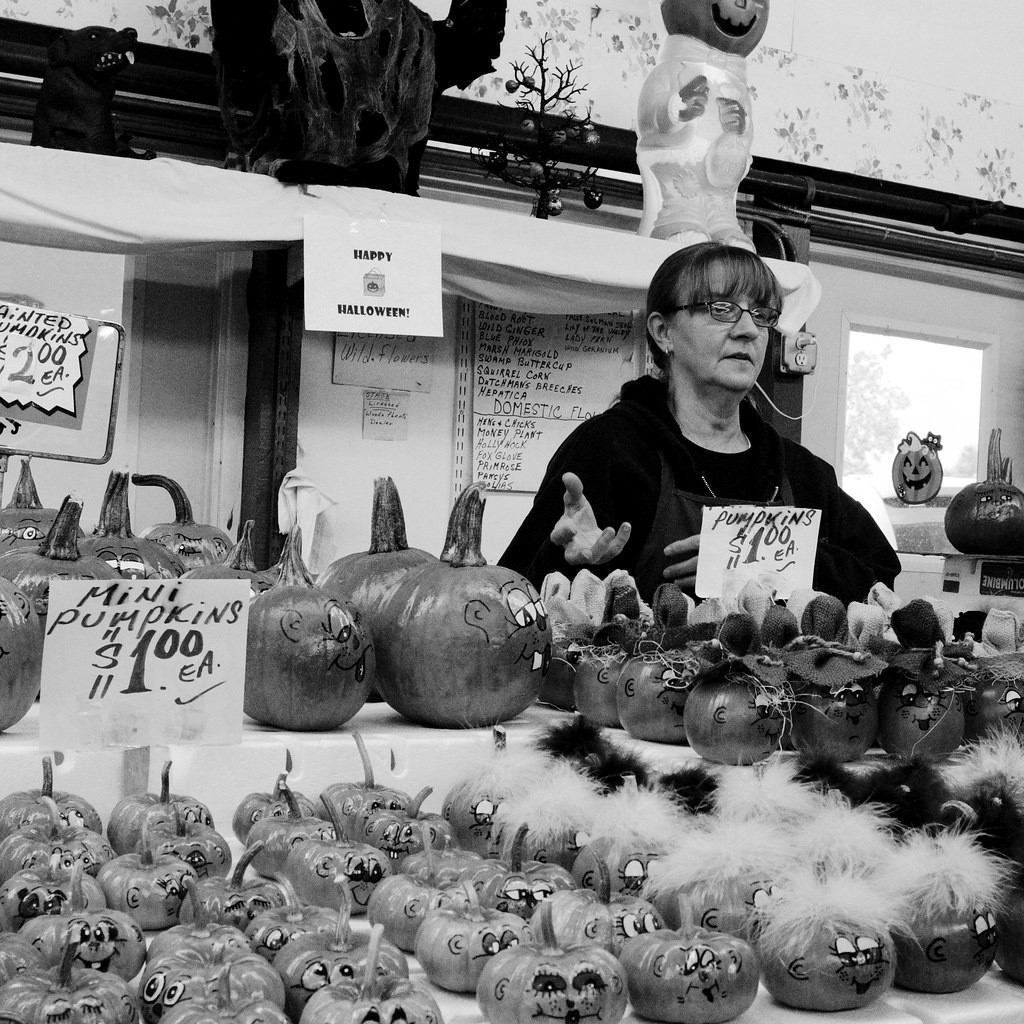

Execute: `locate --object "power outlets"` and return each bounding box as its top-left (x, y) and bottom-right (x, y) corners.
top-left (780, 331), bottom-right (817, 376)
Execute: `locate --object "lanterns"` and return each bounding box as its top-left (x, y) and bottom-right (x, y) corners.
top-left (944, 429), bottom-right (1024, 555)
top-left (0, 457), bottom-right (1024, 1022)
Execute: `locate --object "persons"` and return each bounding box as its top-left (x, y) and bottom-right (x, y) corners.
top-left (493, 240), bottom-right (902, 610)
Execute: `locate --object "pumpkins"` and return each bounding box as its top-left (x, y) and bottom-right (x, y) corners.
top-left (1, 426), bottom-right (1024, 1023)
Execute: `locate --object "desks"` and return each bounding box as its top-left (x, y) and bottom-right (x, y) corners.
top-left (0, 857), bottom-right (1024, 1024)
top-left (0, 702), bottom-right (1023, 857)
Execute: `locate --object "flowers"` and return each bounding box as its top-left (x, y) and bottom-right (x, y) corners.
top-left (975, 148), bottom-right (1018, 203)
top-left (518, 0), bottom-right (585, 65)
top-left (56, 0), bottom-right (80, 19)
top-left (461, 75), bottom-right (503, 102)
top-left (747, 85), bottom-right (760, 104)
top-left (146, 0), bottom-right (215, 50)
top-left (109, 0), bottom-right (119, 26)
top-left (548, 97), bottom-right (602, 122)
top-left (911, 104), bottom-right (963, 181)
top-left (958, 94), bottom-right (995, 121)
top-left (824, 68), bottom-right (894, 136)
top-left (589, 5), bottom-right (660, 81)
top-left (774, 106), bottom-right (823, 165)
top-left (851, 143), bottom-right (908, 184)
top-left (751, 46), bottom-right (799, 78)
top-left (3, 0), bottom-right (30, 19)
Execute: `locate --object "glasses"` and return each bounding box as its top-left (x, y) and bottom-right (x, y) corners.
top-left (661, 300), bottom-right (782, 328)
